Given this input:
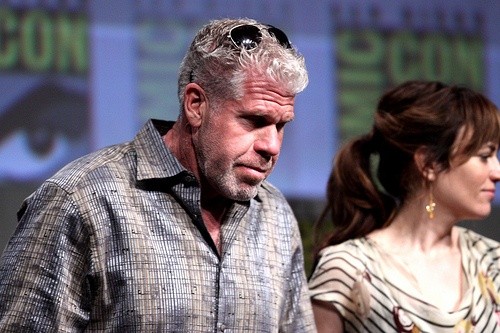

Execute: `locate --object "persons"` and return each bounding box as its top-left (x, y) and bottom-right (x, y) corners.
top-left (307, 77), bottom-right (500, 333)
top-left (0, 14), bottom-right (322, 333)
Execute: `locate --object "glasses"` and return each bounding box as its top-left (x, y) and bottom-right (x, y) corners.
top-left (189, 23), bottom-right (291, 83)
